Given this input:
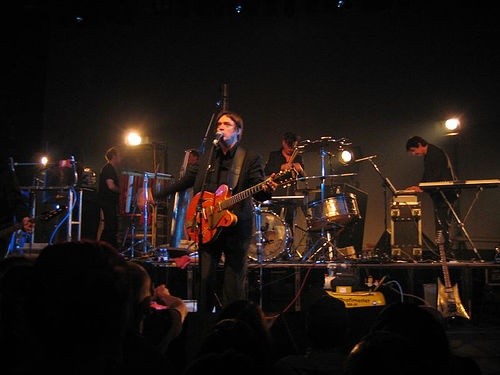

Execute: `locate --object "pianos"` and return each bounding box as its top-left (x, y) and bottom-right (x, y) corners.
top-left (418, 179), bottom-right (500, 260)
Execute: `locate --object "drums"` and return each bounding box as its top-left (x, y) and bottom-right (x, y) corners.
top-left (247, 210), bottom-right (292, 260)
top-left (306, 192), bottom-right (361, 230)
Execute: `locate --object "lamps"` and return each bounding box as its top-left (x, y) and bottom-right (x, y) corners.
top-left (341, 144), bottom-right (359, 163)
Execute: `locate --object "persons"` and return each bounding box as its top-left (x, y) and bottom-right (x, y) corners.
top-left (188, 149), bottom-right (199, 165)
top-left (265, 132), bottom-right (306, 262)
top-left (0, 239), bottom-right (482, 375)
top-left (154, 111), bottom-right (277, 317)
top-left (407, 137), bottom-right (467, 261)
top-left (99, 147), bottom-right (122, 249)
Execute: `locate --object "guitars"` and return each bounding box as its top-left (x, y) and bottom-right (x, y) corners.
top-left (435, 230), bottom-right (471, 319)
top-left (183, 168), bottom-right (298, 244)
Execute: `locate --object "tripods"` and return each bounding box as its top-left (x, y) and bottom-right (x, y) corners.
top-left (121, 174), bottom-right (157, 257)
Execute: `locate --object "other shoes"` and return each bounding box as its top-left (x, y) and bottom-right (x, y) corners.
top-left (433, 257), bottom-right (456, 263)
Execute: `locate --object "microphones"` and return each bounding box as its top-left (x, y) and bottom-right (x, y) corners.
top-left (355, 156), bottom-right (377, 163)
top-left (212, 132), bottom-right (224, 146)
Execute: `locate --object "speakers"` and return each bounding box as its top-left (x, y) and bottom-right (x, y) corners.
top-left (312, 182), bottom-right (368, 258)
